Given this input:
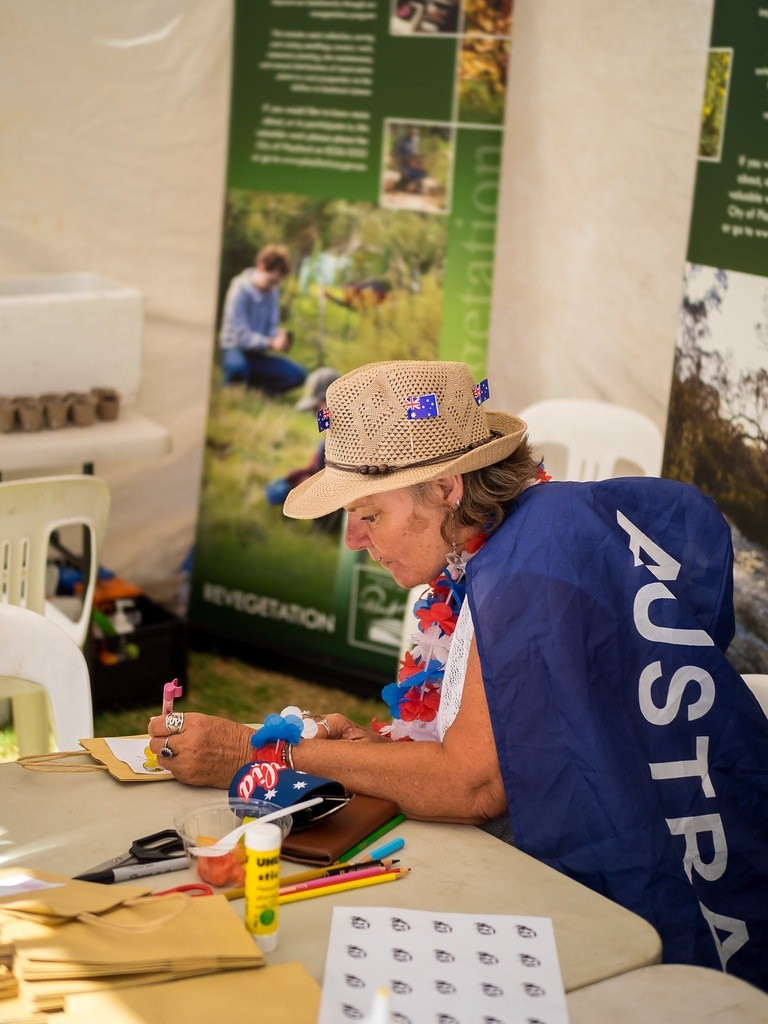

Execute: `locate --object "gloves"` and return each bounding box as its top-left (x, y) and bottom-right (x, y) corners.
top-left (270, 481), bottom-right (290, 504)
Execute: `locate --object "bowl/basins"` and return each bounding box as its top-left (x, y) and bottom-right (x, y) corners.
top-left (176, 798), bottom-right (292, 887)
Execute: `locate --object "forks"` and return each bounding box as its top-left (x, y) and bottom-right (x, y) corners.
top-left (189, 797), bottom-right (324, 857)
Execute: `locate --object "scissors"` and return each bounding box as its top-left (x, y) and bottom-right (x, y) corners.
top-left (72, 827), bottom-right (189, 883)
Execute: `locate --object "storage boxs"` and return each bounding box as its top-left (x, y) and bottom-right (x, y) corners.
top-left (0, 271), bottom-right (141, 409)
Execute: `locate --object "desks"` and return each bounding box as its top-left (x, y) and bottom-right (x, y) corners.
top-left (0, 411), bottom-right (169, 685)
top-left (0, 751), bottom-right (664, 1024)
top-left (567, 964), bottom-right (768, 1024)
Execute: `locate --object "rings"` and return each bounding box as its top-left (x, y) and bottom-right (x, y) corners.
top-left (317, 719), bottom-right (330, 736)
top-left (308, 712), bottom-right (321, 721)
top-left (160, 737), bottom-right (173, 758)
top-left (165, 712), bottom-right (185, 734)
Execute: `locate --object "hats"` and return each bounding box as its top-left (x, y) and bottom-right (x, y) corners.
top-left (295, 366), bottom-right (339, 412)
top-left (281, 360), bottom-right (528, 521)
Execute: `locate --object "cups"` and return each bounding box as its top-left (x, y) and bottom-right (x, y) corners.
top-left (0, 389), bottom-right (121, 432)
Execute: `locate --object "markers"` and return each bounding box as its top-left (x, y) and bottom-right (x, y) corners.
top-left (74, 857), bottom-right (189, 885)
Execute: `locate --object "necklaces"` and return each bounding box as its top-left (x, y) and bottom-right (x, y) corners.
top-left (370, 459), bottom-right (552, 742)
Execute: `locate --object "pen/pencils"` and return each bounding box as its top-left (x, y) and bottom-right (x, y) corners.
top-left (162, 678), bottom-right (183, 714)
top-left (224, 813), bottom-right (411, 905)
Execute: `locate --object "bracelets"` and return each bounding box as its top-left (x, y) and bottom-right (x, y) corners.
top-left (248, 705), bottom-right (319, 771)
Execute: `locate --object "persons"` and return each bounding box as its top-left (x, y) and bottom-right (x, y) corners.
top-left (393, 123), bottom-right (428, 193)
top-left (147, 358), bottom-right (767, 995)
top-left (217, 243), bottom-right (308, 397)
top-left (262, 369), bottom-right (344, 536)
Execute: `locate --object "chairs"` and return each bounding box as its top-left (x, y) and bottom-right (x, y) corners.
top-left (0, 603), bottom-right (95, 764)
top-left (398, 399), bottom-right (663, 688)
top-left (0, 474), bottom-right (111, 651)
